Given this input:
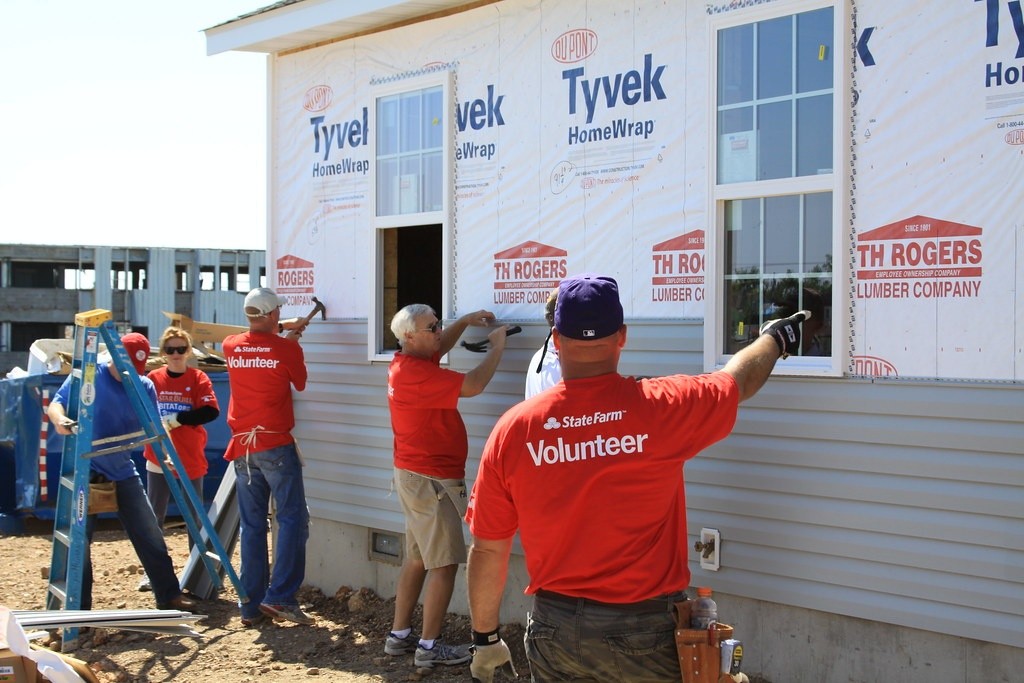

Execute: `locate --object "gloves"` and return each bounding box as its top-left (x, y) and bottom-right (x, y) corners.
top-left (162, 413), bottom-right (181, 431)
top-left (759, 310), bottom-right (812, 360)
top-left (470, 626), bottom-right (519, 683)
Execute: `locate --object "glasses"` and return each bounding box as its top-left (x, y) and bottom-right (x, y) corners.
top-left (414, 319), bottom-right (442, 333)
top-left (163, 346), bottom-right (188, 355)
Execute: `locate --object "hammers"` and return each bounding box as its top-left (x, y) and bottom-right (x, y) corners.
top-left (460, 326), bottom-right (522, 352)
top-left (296, 297), bottom-right (326, 334)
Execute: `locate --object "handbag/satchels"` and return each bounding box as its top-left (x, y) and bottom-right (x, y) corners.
top-left (669, 600), bottom-right (736, 683)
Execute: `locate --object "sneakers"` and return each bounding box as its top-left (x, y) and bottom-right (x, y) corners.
top-left (413, 635), bottom-right (475, 667)
top-left (383, 627), bottom-right (422, 655)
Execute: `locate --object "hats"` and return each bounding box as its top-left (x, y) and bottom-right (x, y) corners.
top-left (243, 287), bottom-right (286, 318)
top-left (554, 273), bottom-right (623, 340)
top-left (120, 333), bottom-right (151, 375)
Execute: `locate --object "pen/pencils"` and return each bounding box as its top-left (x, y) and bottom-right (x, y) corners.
top-left (709, 624), bottom-right (714, 646)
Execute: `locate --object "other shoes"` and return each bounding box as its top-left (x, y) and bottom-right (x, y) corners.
top-left (240, 612), bottom-right (264, 625)
top-left (138, 569), bottom-right (153, 591)
top-left (157, 594), bottom-right (196, 609)
top-left (258, 602), bottom-right (316, 625)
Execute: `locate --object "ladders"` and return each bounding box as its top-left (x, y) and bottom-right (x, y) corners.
top-left (45, 308), bottom-right (250, 652)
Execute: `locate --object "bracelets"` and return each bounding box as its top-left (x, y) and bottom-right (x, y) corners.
top-left (278, 323), bottom-right (284, 334)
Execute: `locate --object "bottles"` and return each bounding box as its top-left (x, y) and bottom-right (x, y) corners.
top-left (691, 588), bottom-right (718, 629)
top-left (734, 307), bottom-right (745, 340)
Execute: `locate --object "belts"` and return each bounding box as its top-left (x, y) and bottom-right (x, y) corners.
top-left (536, 587), bottom-right (683, 609)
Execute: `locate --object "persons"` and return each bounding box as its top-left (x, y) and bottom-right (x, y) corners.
top-left (222, 288), bottom-right (315, 626)
top-left (47, 333), bottom-right (197, 646)
top-left (384, 304), bottom-right (508, 668)
top-left (525, 286), bottom-right (563, 399)
top-left (737, 279), bottom-right (824, 356)
top-left (139, 327), bottom-right (221, 591)
top-left (468, 274), bottom-right (812, 683)
